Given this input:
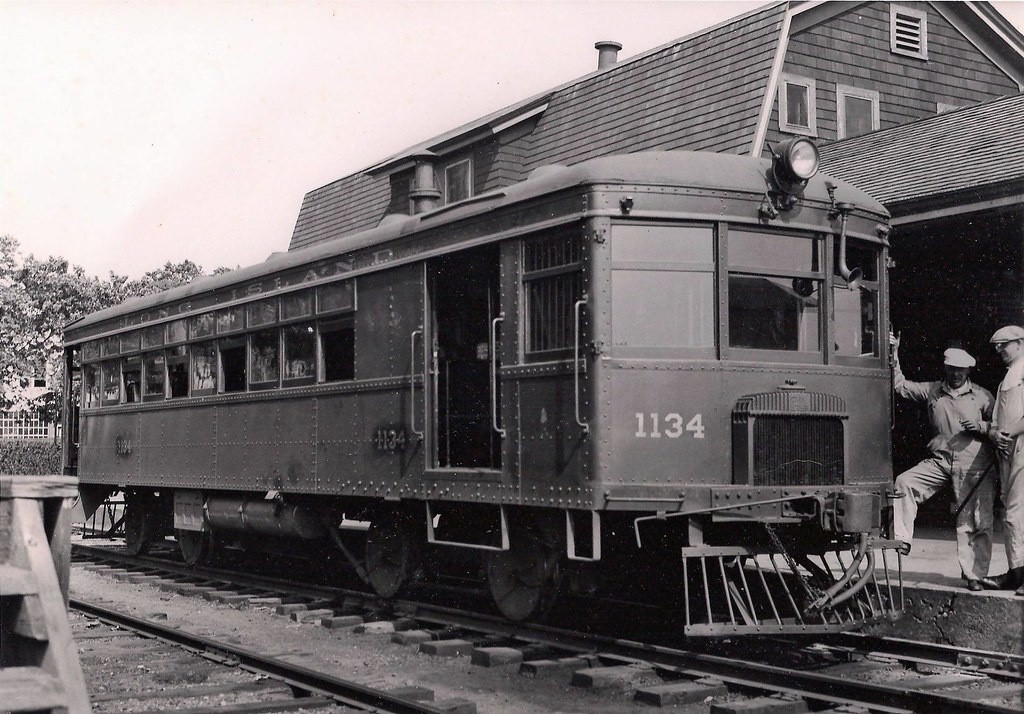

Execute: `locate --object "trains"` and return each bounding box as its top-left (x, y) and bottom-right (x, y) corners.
top-left (61, 138), bottom-right (910, 636)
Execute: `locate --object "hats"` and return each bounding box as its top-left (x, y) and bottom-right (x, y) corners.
top-left (944, 348), bottom-right (976, 369)
top-left (990, 326), bottom-right (1024, 343)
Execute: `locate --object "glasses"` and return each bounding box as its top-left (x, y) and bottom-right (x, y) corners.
top-left (995, 343), bottom-right (1006, 351)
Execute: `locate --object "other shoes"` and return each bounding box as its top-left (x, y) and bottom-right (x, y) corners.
top-left (968, 580), bottom-right (983, 591)
top-left (983, 567), bottom-right (1024, 595)
top-left (882, 531), bottom-right (910, 556)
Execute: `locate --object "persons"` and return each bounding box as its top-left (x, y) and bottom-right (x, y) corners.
top-left (886, 330), bottom-right (992, 591)
top-left (988, 325), bottom-right (1023, 597)
